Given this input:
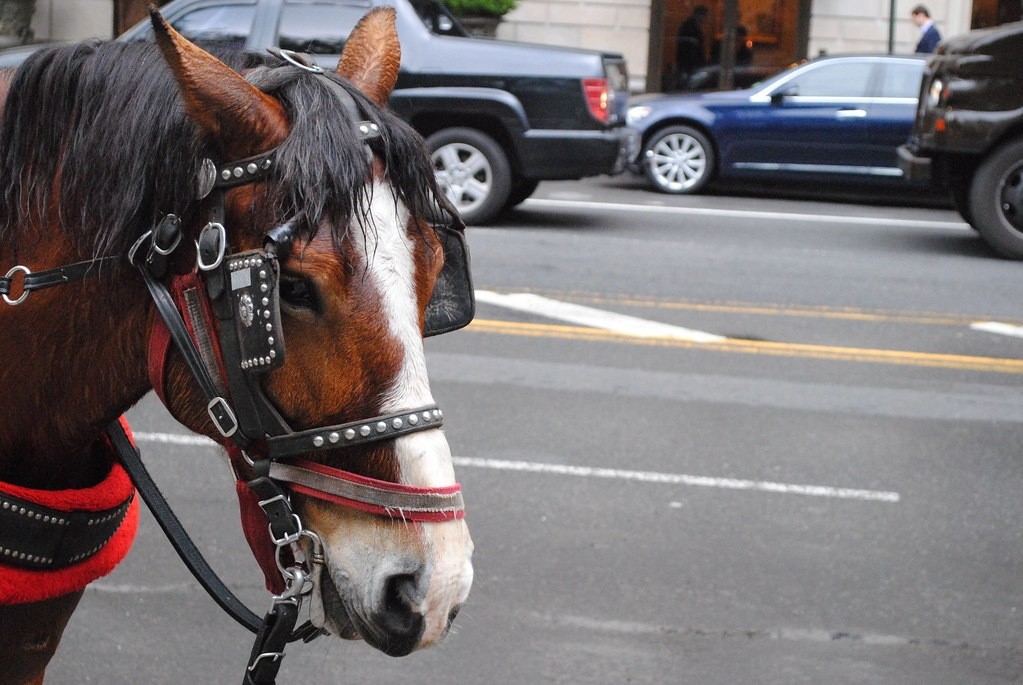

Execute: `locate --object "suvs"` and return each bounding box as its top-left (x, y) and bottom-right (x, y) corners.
top-left (897, 18), bottom-right (1022, 260)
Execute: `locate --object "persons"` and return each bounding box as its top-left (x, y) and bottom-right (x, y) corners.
top-left (712, 25), bottom-right (752, 88)
top-left (912, 6), bottom-right (940, 53)
top-left (674, 4), bottom-right (708, 93)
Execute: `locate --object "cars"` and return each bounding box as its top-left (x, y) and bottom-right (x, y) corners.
top-left (628, 54), bottom-right (954, 194)
top-left (0, 1), bottom-right (641, 225)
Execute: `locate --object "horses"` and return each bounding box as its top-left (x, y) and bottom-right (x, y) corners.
top-left (0, 1), bottom-right (474, 685)
